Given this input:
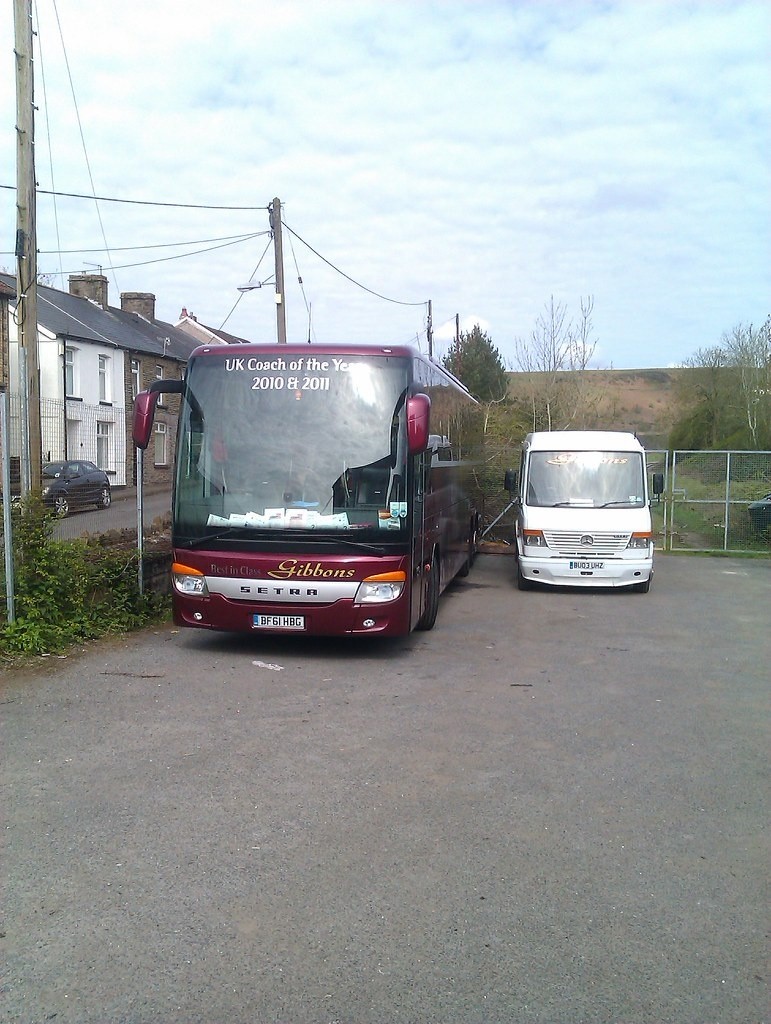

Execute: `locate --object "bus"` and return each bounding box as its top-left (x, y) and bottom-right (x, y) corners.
top-left (126, 342), bottom-right (489, 639)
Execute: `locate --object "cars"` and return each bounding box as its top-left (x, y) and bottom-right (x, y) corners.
top-left (37, 459), bottom-right (111, 518)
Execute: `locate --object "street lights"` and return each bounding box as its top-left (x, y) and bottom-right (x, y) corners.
top-left (235, 275), bottom-right (286, 343)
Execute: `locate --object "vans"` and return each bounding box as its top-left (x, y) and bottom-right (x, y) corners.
top-left (512, 427), bottom-right (657, 594)
